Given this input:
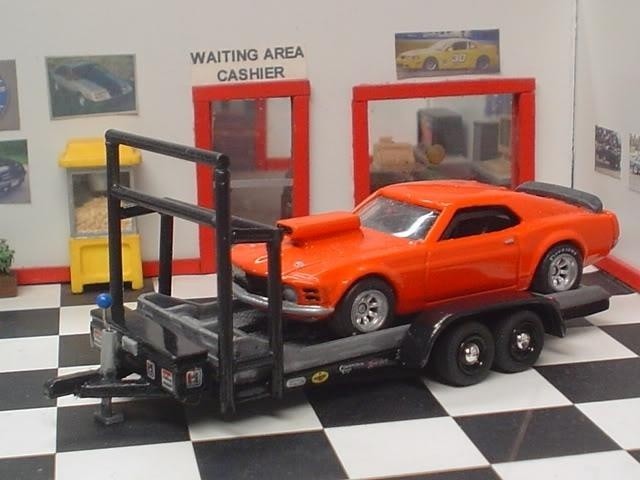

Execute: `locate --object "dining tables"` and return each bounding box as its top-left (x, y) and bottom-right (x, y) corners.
top-left (0, 238), bottom-right (19, 300)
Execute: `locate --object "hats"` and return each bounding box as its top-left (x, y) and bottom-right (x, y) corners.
top-left (53, 60), bottom-right (133, 107)
top-left (0, 158), bottom-right (25, 193)
top-left (397, 37), bottom-right (498, 71)
